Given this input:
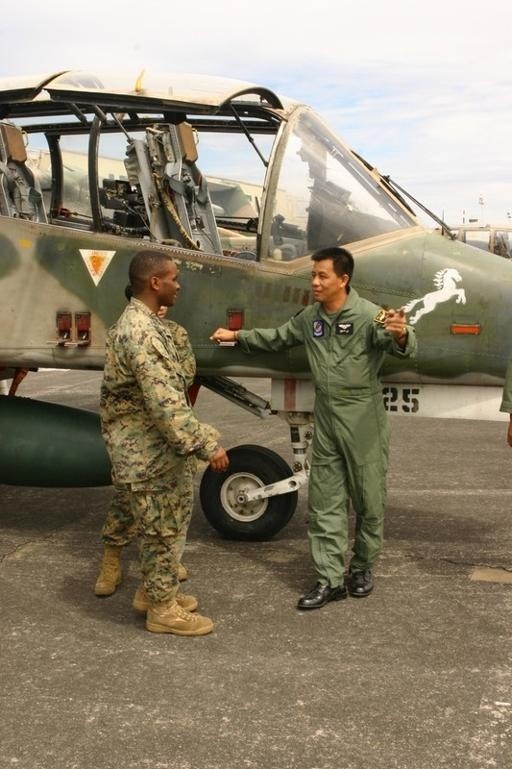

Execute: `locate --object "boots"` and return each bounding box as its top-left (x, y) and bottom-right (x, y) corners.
top-left (94, 548), bottom-right (215, 635)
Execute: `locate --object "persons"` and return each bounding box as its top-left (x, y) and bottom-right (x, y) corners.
top-left (94, 284), bottom-right (198, 597)
top-left (208, 246), bottom-right (418, 611)
top-left (98, 251), bottom-right (231, 637)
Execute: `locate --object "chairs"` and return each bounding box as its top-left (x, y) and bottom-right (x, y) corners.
top-left (145, 120), bottom-right (228, 257)
top-left (2, 122), bottom-right (48, 224)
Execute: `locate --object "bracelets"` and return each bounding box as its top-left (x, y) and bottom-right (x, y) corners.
top-left (233, 330), bottom-right (239, 341)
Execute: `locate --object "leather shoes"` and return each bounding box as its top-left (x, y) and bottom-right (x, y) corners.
top-left (296, 582), bottom-right (348, 610)
top-left (347, 569), bottom-right (374, 596)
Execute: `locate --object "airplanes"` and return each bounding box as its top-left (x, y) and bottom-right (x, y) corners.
top-left (2, 71), bottom-right (512, 542)
top-left (25, 142), bottom-right (512, 260)
